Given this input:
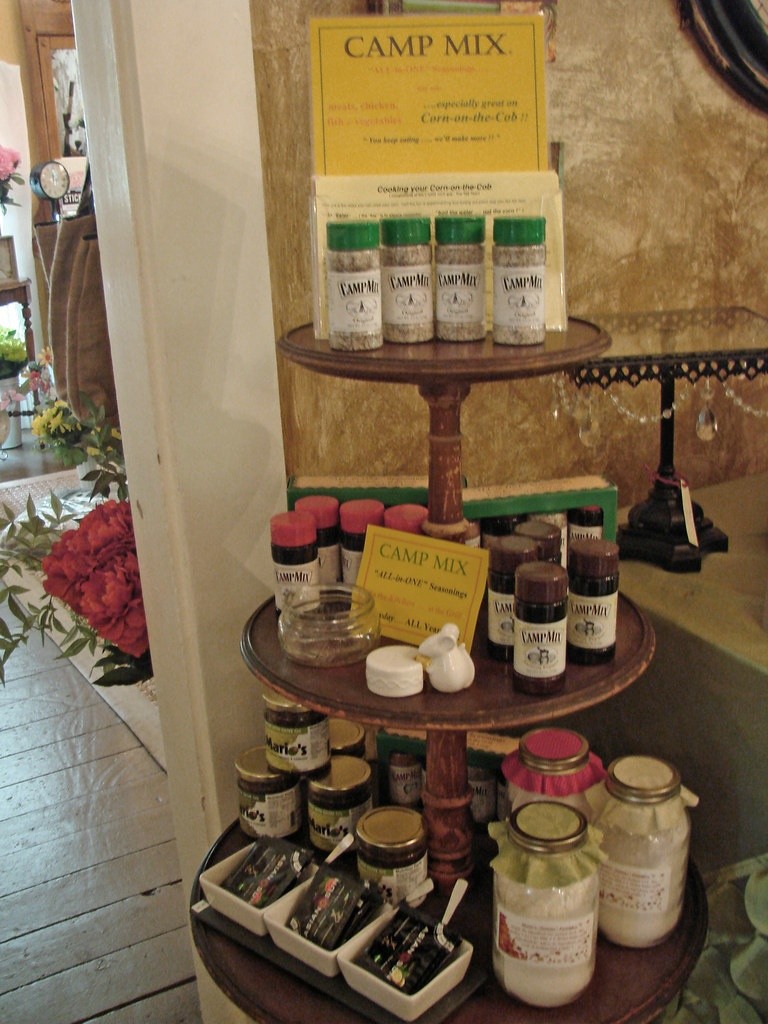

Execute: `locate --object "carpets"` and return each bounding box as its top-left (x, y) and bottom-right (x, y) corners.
top-left (2, 471), bottom-right (167, 769)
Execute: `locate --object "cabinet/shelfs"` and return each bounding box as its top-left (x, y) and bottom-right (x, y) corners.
top-left (180, 312), bottom-right (706, 1024)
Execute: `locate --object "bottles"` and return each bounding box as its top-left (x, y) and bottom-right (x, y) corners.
top-left (567, 539), bottom-right (618, 667)
top-left (488, 800), bottom-right (609, 1007)
top-left (487, 536), bottom-right (537, 663)
top-left (235, 747), bottom-right (304, 843)
top-left (340, 499), bottom-right (384, 585)
top-left (480, 507), bottom-right (603, 570)
top-left (326, 220), bottom-right (383, 351)
top-left (295, 495), bottom-right (342, 587)
top-left (389, 751), bottom-right (509, 822)
top-left (513, 561), bottom-right (569, 697)
top-left (357, 807), bottom-right (429, 910)
top-left (279, 583), bottom-right (381, 667)
top-left (271, 511), bottom-right (321, 620)
top-left (492, 217), bottom-right (546, 346)
top-left (262, 686), bottom-right (331, 776)
top-left (385, 504), bottom-right (427, 538)
top-left (328, 718), bottom-right (366, 762)
top-left (380, 218), bottom-right (435, 344)
top-left (502, 727), bottom-right (606, 831)
top-left (308, 755), bottom-right (375, 854)
top-left (435, 216), bottom-right (486, 341)
top-left (584, 754), bottom-right (698, 948)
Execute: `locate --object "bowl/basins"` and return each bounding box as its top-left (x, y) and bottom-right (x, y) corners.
top-left (337, 906), bottom-right (473, 1021)
top-left (200, 843), bottom-right (320, 936)
top-left (263, 877), bottom-right (393, 977)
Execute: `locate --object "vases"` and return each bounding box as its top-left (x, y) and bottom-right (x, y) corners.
top-left (0, 373), bottom-right (23, 448)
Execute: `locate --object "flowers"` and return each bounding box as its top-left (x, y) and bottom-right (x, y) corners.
top-left (0, 323), bottom-right (158, 690)
top-left (0, 140), bottom-right (25, 215)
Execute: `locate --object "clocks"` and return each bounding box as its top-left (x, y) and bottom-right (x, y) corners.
top-left (29, 159), bottom-right (71, 199)
